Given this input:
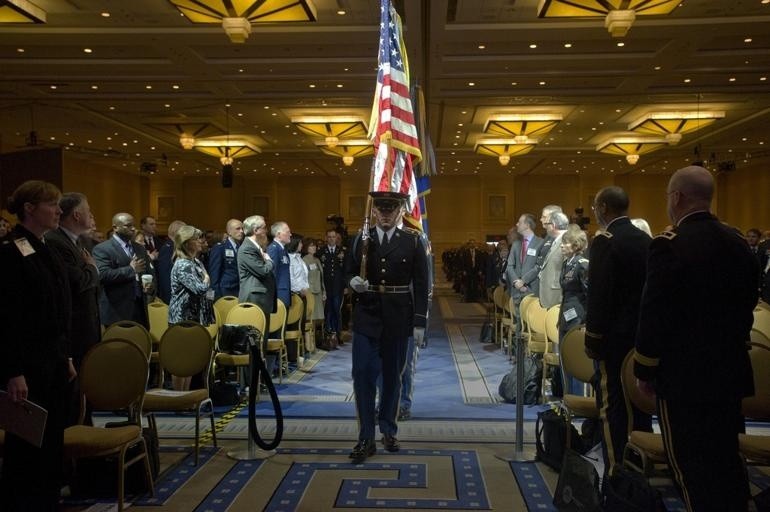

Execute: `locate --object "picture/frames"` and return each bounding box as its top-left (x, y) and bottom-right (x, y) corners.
top-left (250, 193), bottom-right (272, 221)
top-left (155, 195), bottom-right (177, 221)
top-left (488, 193), bottom-right (509, 221)
top-left (347, 194), bottom-right (367, 221)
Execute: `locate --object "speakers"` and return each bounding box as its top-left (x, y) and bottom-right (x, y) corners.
top-left (221, 166), bottom-right (233, 188)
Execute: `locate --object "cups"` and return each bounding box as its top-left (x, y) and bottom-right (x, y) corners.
top-left (141, 274), bottom-right (154, 292)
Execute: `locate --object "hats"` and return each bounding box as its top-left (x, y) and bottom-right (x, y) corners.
top-left (367, 190), bottom-right (411, 211)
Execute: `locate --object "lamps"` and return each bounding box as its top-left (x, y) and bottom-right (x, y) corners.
top-left (628, 111), bottom-right (727, 146)
top-left (195, 140), bottom-right (262, 167)
top-left (474, 139), bottom-right (538, 167)
top-left (173, 0), bottom-right (318, 46)
top-left (142, 115), bottom-right (226, 150)
top-left (314, 140), bottom-right (374, 166)
top-left (0, 0), bottom-right (47, 25)
top-left (483, 114), bottom-right (564, 144)
top-left (291, 116), bottom-right (369, 149)
top-left (595, 137), bottom-right (668, 165)
top-left (536, 0), bottom-right (684, 38)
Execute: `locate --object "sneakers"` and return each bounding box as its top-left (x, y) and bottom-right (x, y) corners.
top-left (381, 436), bottom-right (399, 452)
top-left (372, 402), bottom-right (382, 425)
top-left (479, 320), bottom-right (495, 345)
top-left (348, 438), bottom-right (376, 459)
top-left (499, 352), bottom-right (543, 405)
top-left (397, 406), bottom-right (411, 421)
top-left (536, 406), bottom-right (601, 464)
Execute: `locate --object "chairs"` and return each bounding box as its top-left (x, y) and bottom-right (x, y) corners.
top-left (147, 302), bottom-right (170, 384)
top-left (61, 338), bottom-right (155, 512)
top-left (101, 319), bottom-right (155, 447)
top-left (202, 290), bottom-right (316, 402)
top-left (142, 320), bottom-right (217, 468)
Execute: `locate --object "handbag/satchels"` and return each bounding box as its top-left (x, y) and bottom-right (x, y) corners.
top-left (210, 377), bottom-right (242, 407)
top-left (305, 330), bottom-right (315, 353)
top-left (315, 322), bottom-right (339, 350)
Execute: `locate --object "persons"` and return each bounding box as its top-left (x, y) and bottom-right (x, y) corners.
top-left (238, 215), bottom-right (275, 392)
top-left (93, 211), bottom-right (155, 332)
top-left (393, 192), bottom-right (435, 422)
top-left (2, 180), bottom-right (78, 511)
top-left (342, 189), bottom-right (428, 459)
top-left (442, 200), bottom-right (590, 398)
top-left (46, 191), bottom-right (102, 426)
top-left (168, 226), bottom-right (210, 412)
top-left (584, 184), bottom-right (655, 479)
top-left (2, 198), bottom-right (112, 264)
top-left (132, 213), bottom-right (189, 307)
top-left (633, 167), bottom-right (760, 509)
top-left (201, 216), bottom-right (351, 363)
top-left (632, 215), bottom-right (770, 310)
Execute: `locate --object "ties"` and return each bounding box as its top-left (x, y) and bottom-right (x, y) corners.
top-left (126, 243), bottom-right (142, 297)
top-left (521, 238), bottom-right (527, 265)
top-left (148, 238), bottom-right (155, 250)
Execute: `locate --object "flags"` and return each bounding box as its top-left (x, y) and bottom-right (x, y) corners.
top-left (368, 1), bottom-right (432, 233)
